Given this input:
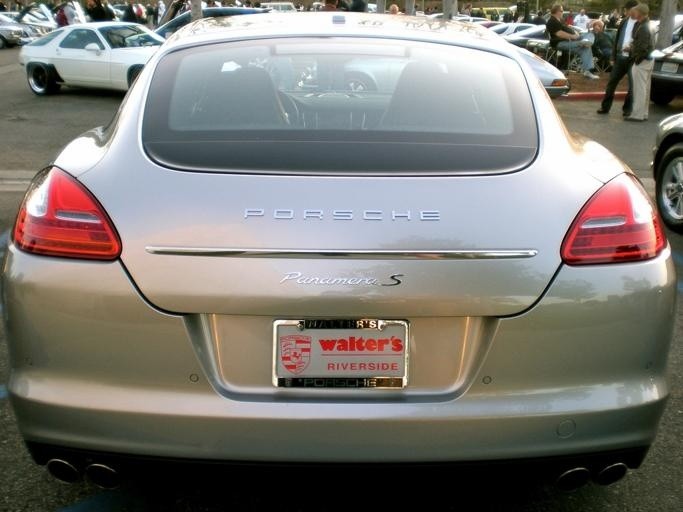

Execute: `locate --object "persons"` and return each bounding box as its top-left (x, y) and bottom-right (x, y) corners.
top-left (412, 1), bottom-right (657, 123)
top-left (52, 0), bottom-right (405, 30)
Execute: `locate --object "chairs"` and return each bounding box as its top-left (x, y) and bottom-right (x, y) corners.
top-left (544, 30), bottom-right (617, 74)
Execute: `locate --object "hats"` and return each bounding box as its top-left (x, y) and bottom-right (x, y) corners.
top-left (632, 3), bottom-right (650, 14)
top-left (623, 0), bottom-right (640, 10)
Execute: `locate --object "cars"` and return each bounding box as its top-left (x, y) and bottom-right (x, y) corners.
top-left (2, 11), bottom-right (674, 494)
top-left (650, 110), bottom-right (682, 235)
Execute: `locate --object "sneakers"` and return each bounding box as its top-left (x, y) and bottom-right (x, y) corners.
top-left (620, 110), bottom-right (649, 123)
top-left (596, 107), bottom-right (610, 115)
top-left (582, 69), bottom-right (600, 81)
top-left (576, 39), bottom-right (593, 49)
